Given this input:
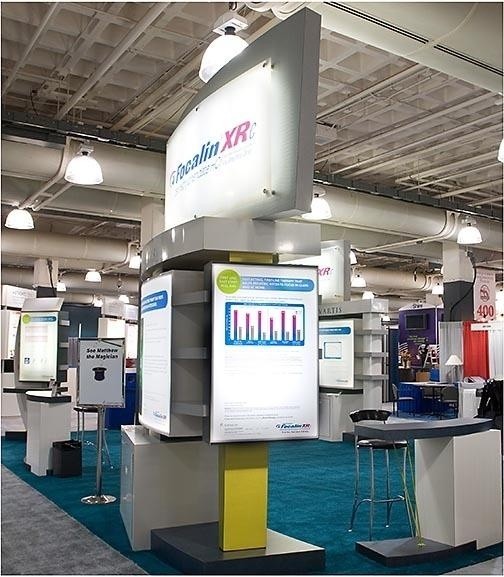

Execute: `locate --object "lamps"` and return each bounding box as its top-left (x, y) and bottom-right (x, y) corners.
top-left (4, 200), bottom-right (39, 229)
top-left (302, 182), bottom-right (331, 221)
top-left (57, 251), bottom-right (142, 308)
top-left (455, 213), bottom-right (487, 245)
top-left (197, 6), bottom-right (252, 83)
top-left (350, 247), bottom-right (446, 321)
top-left (61, 108), bottom-right (106, 186)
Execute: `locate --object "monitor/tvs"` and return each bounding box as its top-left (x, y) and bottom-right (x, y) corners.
top-left (404, 314), bottom-right (427, 330)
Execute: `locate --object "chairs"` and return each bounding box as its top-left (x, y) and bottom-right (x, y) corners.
top-left (348, 408), bottom-right (419, 542)
top-left (73, 404), bottom-right (107, 463)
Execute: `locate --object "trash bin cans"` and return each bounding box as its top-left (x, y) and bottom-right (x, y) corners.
top-left (52, 440), bottom-right (82, 477)
